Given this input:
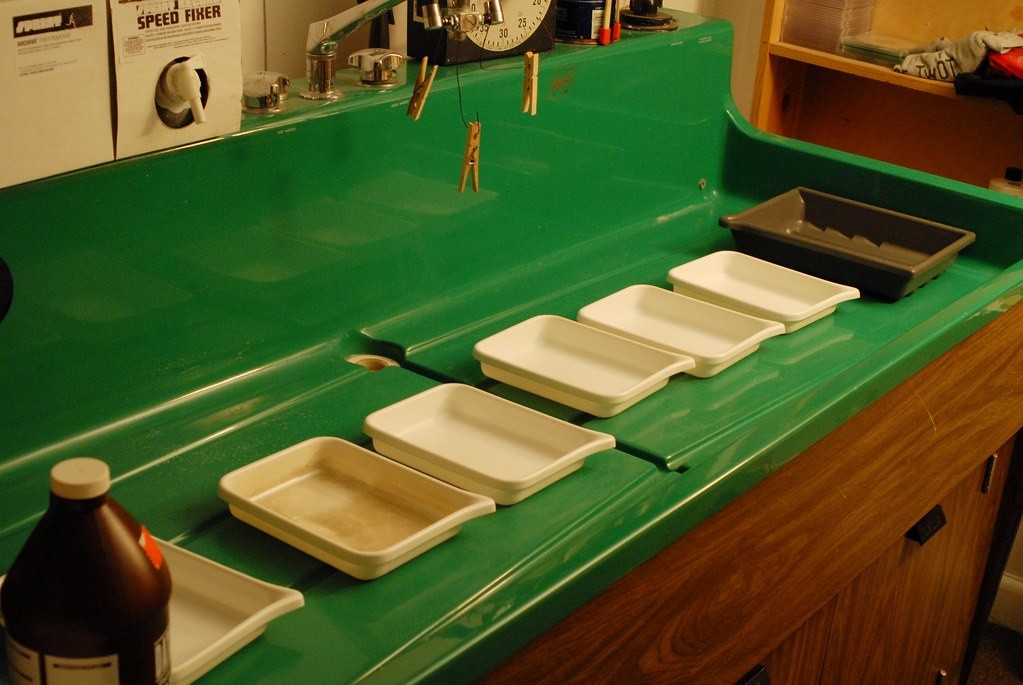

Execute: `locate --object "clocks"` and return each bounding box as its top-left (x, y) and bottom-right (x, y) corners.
top-left (444, 0), bottom-right (558, 60)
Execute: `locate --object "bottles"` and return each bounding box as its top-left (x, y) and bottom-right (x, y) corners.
top-left (0, 457), bottom-right (172, 685)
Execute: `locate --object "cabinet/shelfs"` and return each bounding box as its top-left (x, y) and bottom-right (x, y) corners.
top-left (473, 302), bottom-right (1023, 685)
top-left (748, 1), bottom-right (1023, 196)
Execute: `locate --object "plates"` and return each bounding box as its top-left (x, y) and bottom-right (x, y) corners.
top-left (156, 189), bottom-right (979, 685)
